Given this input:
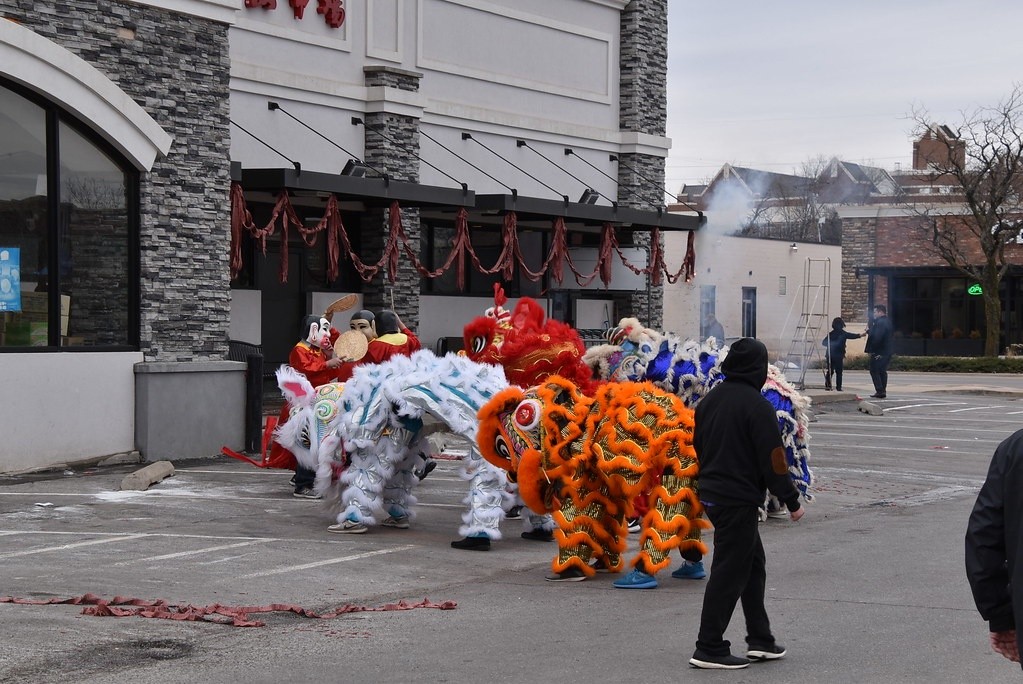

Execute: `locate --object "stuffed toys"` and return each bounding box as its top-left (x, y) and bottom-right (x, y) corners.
top-left (475, 373), bottom-right (715, 588)
top-left (578, 317), bottom-right (816, 520)
top-left (271, 349), bottom-right (559, 550)
top-left (459, 297), bottom-right (615, 402)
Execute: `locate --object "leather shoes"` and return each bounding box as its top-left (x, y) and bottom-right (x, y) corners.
top-left (870, 391), bottom-right (886, 397)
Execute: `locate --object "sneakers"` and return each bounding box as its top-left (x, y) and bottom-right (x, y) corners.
top-left (451, 537), bottom-right (491, 551)
top-left (414, 458), bottom-right (436, 481)
top-left (689, 649), bottom-right (751, 668)
top-left (746, 645), bottom-right (786, 659)
top-left (290, 476), bottom-right (296, 485)
top-left (590, 560), bottom-right (613, 571)
top-left (545, 565), bottom-right (588, 582)
top-left (672, 561), bottom-right (707, 579)
top-left (504, 506), bottom-right (523, 520)
top-left (327, 520), bottom-right (369, 534)
top-left (293, 487), bottom-right (322, 499)
top-left (521, 528), bottom-right (555, 541)
top-left (380, 515), bottom-right (410, 528)
top-left (612, 569), bottom-right (658, 588)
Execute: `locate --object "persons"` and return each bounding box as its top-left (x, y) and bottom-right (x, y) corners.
top-left (321, 309), bottom-right (378, 467)
top-left (864, 304), bottom-right (894, 398)
top-left (357, 309), bottom-right (436, 483)
top-left (822, 316), bottom-right (868, 392)
top-left (965, 426), bottom-right (1023, 672)
top-left (290, 314), bottom-right (349, 500)
top-left (690, 338), bottom-right (806, 669)
top-left (699, 313), bottom-right (726, 350)
